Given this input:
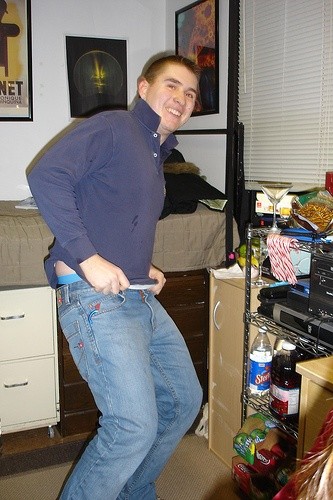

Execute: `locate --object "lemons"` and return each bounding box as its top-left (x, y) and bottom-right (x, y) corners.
top-left (238, 243), bottom-right (259, 268)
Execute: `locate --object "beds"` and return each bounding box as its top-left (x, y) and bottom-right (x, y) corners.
top-left (0, 121), bottom-right (248, 291)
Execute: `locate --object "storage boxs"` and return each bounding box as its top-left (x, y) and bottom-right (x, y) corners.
top-left (231, 413), bottom-right (295, 500)
top-left (256, 191), bottom-right (298, 217)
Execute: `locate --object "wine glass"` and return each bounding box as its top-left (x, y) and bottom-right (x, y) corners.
top-left (262, 184), bottom-right (292, 232)
top-left (252, 231), bottom-right (270, 287)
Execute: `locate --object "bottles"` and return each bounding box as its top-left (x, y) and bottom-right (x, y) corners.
top-left (248, 326), bottom-right (272, 395)
top-left (270, 342), bottom-right (303, 417)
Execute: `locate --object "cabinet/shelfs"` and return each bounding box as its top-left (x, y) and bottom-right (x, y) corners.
top-left (59, 261), bottom-right (210, 438)
top-left (239, 226), bottom-right (333, 457)
top-left (199, 274), bottom-right (276, 472)
top-left (0, 283), bottom-right (58, 435)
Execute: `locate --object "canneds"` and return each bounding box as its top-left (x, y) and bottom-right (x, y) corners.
top-left (235, 428), bottom-right (296, 487)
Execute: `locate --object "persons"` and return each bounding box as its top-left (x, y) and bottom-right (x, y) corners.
top-left (27, 54), bottom-right (204, 500)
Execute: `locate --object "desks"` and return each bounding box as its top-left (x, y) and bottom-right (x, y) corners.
top-left (294, 354), bottom-right (333, 467)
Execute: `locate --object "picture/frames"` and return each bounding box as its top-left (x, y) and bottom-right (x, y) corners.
top-left (173, 0), bottom-right (221, 118)
top-left (0, 0), bottom-right (34, 121)
top-left (65, 35), bottom-right (128, 118)
